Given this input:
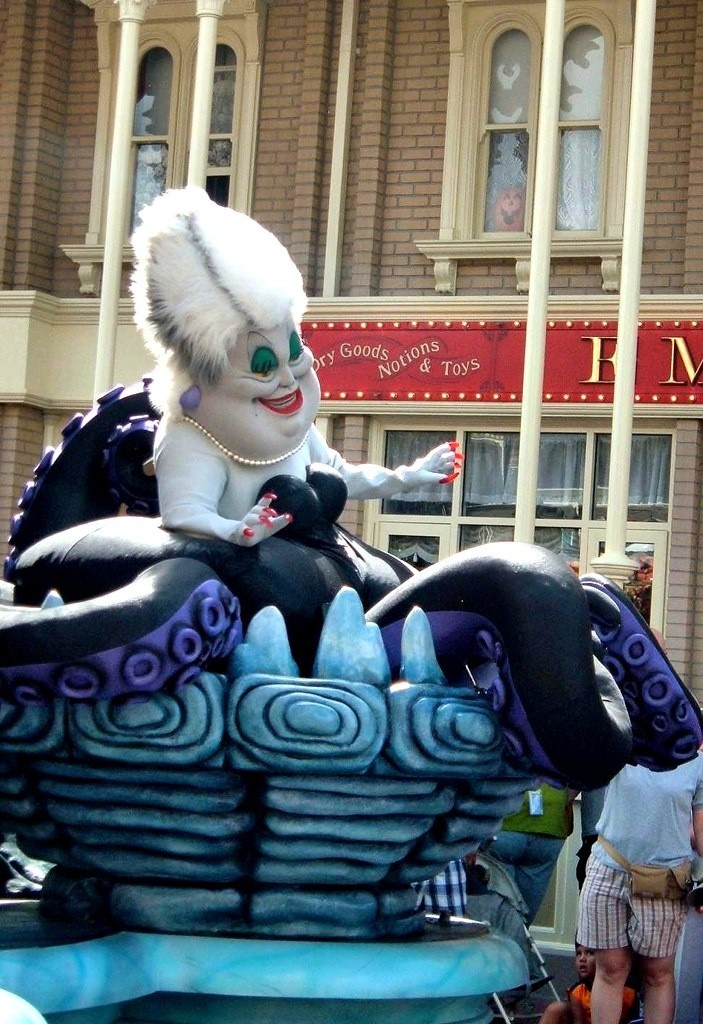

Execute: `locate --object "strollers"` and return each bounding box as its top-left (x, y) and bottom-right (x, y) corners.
top-left (463, 850), bottom-right (561, 1024)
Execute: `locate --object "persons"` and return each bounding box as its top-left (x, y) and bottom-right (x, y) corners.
top-left (130, 185), bottom-right (466, 548)
top-left (410, 740), bottom-right (703, 1024)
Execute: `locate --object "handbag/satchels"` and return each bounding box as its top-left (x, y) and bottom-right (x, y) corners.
top-left (598, 835), bottom-right (692, 900)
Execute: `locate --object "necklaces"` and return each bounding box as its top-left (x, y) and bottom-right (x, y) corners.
top-left (178, 413), bottom-right (313, 466)
